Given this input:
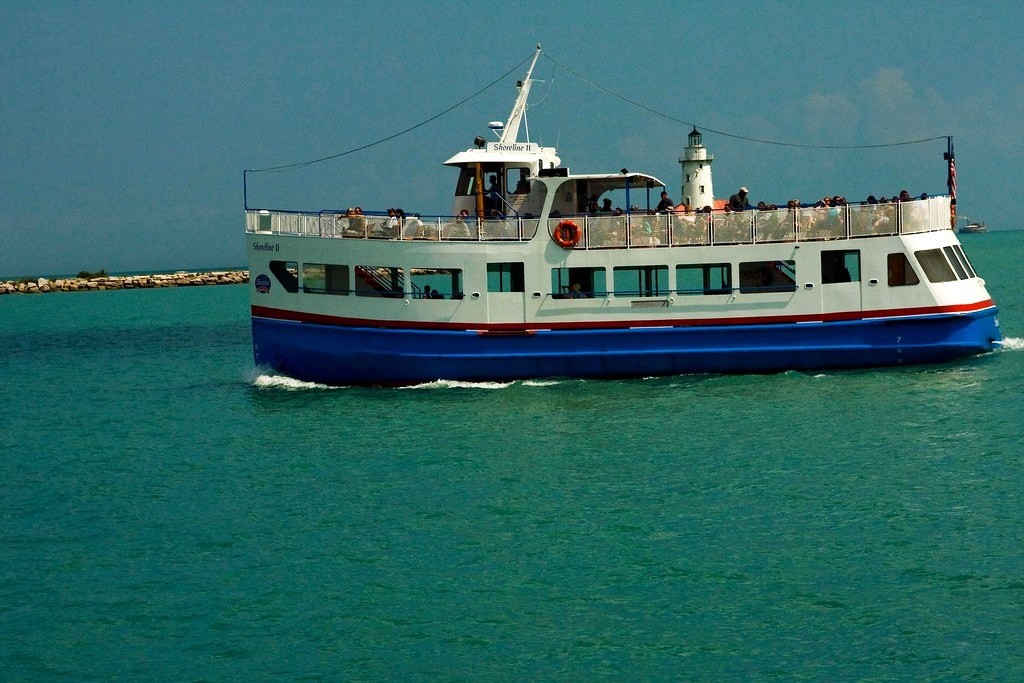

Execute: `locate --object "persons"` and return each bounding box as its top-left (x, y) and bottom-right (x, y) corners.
top-left (591, 191), bottom-right (715, 244)
top-left (518, 213), bottom-right (537, 241)
top-left (549, 210), bottom-right (562, 218)
top-left (484, 175), bottom-right (500, 208)
top-left (422, 285), bottom-right (444, 299)
top-left (396, 286), bottom-right (403, 298)
top-left (337, 206), bottom-right (425, 237)
top-left (485, 210), bottom-right (504, 241)
top-left (449, 209), bottom-right (470, 241)
top-left (860, 190), bottom-right (931, 233)
top-left (562, 284), bottom-right (586, 299)
top-left (720, 187), bottom-right (804, 240)
top-left (807, 196), bottom-right (859, 237)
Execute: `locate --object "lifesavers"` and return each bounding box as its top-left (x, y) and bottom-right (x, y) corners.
top-left (554, 219), bottom-right (580, 248)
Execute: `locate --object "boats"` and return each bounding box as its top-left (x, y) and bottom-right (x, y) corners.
top-left (959, 216), bottom-right (988, 233)
top-left (242, 42), bottom-right (1004, 390)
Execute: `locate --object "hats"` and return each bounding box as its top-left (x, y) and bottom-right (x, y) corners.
top-left (387, 208), bottom-right (396, 214)
top-left (739, 187), bottom-right (749, 193)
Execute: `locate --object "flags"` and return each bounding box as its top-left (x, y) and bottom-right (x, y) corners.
top-left (947, 141), bottom-right (958, 226)
